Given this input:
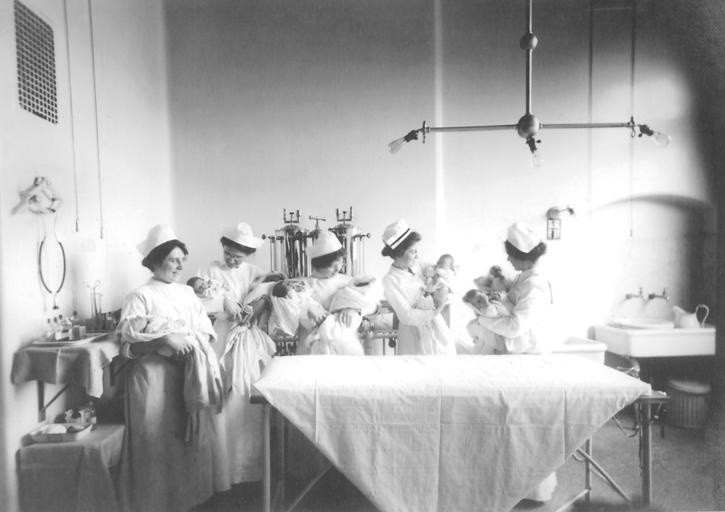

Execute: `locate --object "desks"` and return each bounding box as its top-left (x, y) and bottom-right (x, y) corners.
top-left (25, 332), bottom-right (127, 423)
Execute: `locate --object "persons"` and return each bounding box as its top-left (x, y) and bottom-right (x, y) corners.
top-left (273, 279), bottom-right (304, 298)
top-left (122, 314), bottom-right (203, 360)
top-left (329, 277), bottom-right (383, 328)
top-left (115, 239), bottom-right (232, 512)
top-left (423, 253), bottom-right (455, 310)
top-left (478, 223), bottom-right (557, 503)
top-left (297, 230), bottom-right (354, 356)
top-left (382, 220), bottom-right (456, 357)
top-left (464, 288), bottom-right (513, 318)
top-left (198, 221), bottom-right (277, 493)
top-left (473, 264), bottom-right (513, 295)
top-left (242, 272), bottom-right (286, 318)
top-left (186, 276), bottom-right (207, 294)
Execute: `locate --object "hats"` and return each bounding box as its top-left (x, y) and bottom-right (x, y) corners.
top-left (223, 222), bottom-right (259, 249)
top-left (139, 223), bottom-right (180, 257)
top-left (383, 219), bottom-right (412, 249)
top-left (310, 230), bottom-right (343, 258)
top-left (508, 223), bottom-right (542, 253)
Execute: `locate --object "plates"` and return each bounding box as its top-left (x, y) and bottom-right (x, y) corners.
top-left (28, 424), bottom-right (94, 444)
top-left (31, 332), bottom-right (111, 350)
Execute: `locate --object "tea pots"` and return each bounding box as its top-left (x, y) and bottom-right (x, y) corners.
top-left (671, 303), bottom-right (710, 330)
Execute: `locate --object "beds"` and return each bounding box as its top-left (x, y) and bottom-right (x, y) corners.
top-left (249, 354), bottom-right (672, 511)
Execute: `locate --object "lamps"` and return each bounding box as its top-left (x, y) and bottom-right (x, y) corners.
top-left (638, 120), bottom-right (673, 147)
top-left (388, 126), bottom-right (421, 152)
top-left (545, 205), bottom-right (575, 240)
top-left (527, 138), bottom-right (552, 177)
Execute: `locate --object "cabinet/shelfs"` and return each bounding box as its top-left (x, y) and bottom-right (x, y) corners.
top-left (18, 421), bottom-right (124, 512)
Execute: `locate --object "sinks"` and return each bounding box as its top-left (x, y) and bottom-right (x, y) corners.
top-left (594, 321), bottom-right (716, 358)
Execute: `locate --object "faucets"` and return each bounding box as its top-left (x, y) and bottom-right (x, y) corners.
top-left (625, 287), bottom-right (646, 302)
top-left (648, 288), bottom-right (670, 303)
